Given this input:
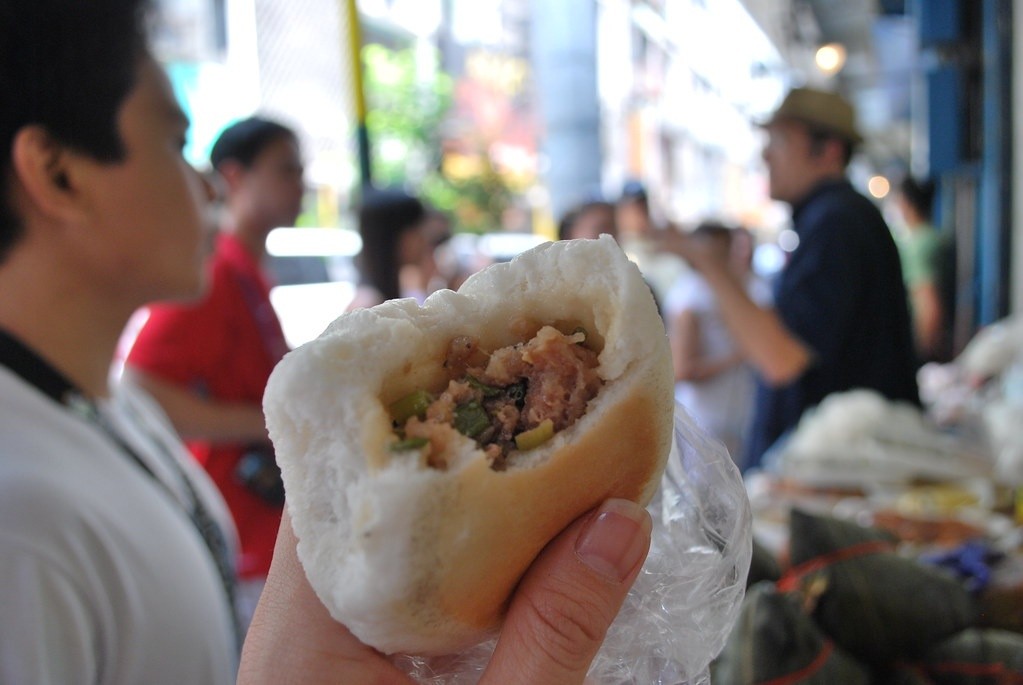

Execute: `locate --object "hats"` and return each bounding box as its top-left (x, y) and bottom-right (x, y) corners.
top-left (757, 86), bottom-right (868, 151)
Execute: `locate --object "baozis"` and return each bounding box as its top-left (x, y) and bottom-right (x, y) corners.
top-left (263, 234), bottom-right (672, 655)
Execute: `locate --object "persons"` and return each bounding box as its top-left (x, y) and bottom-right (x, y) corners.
top-left (236, 494), bottom-right (652, 685)
top-left (0, 0), bottom-right (244, 685)
top-left (338, 183), bottom-right (492, 316)
top-left (559, 171), bottom-right (772, 510)
top-left (889, 172), bottom-right (949, 366)
top-left (653, 87), bottom-right (922, 449)
top-left (121, 115), bottom-right (294, 644)
